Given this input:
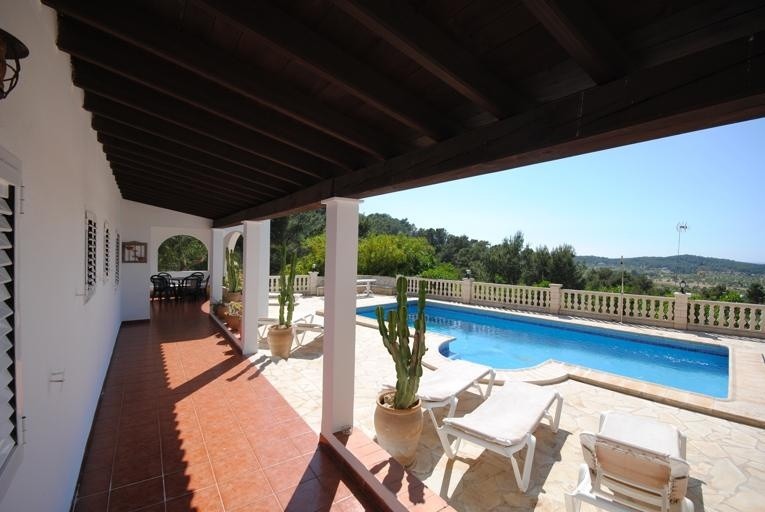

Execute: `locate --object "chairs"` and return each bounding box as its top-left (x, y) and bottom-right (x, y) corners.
top-left (436, 376), bottom-right (564, 494)
top-left (256, 314), bottom-right (324, 353)
top-left (383, 361), bottom-right (496, 445)
top-left (565, 403), bottom-right (689, 511)
top-left (149, 269), bottom-right (210, 303)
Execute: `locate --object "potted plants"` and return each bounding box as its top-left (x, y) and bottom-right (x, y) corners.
top-left (371, 275), bottom-right (433, 472)
top-left (213, 247), bottom-right (242, 338)
top-left (266, 240), bottom-right (301, 360)
